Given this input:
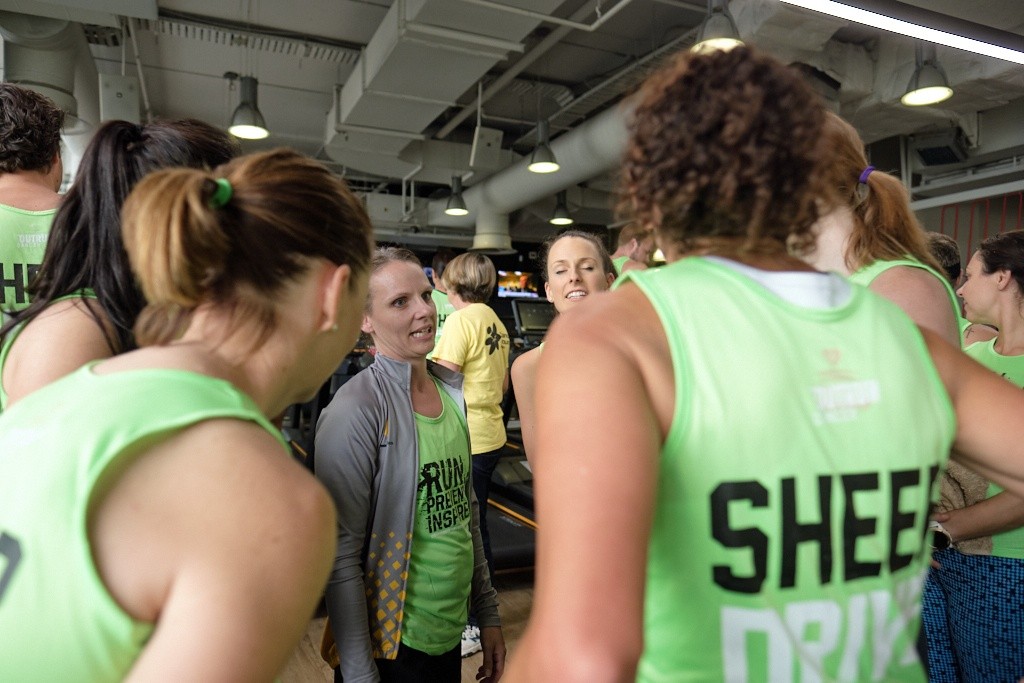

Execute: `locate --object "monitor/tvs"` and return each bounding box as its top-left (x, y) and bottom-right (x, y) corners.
top-left (511, 298), bottom-right (560, 334)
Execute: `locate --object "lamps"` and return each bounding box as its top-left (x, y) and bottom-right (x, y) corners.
top-left (228, 0), bottom-right (270, 140)
top-left (443, 108), bottom-right (470, 216)
top-left (690, 0), bottom-right (746, 54)
top-left (528, 58), bottom-right (560, 174)
top-left (549, 189), bottom-right (575, 226)
top-left (899, 40), bottom-right (953, 106)
top-left (781, 0), bottom-right (1024, 66)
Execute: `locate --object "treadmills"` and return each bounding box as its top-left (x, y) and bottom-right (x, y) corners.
top-left (486, 487), bottom-right (536, 579)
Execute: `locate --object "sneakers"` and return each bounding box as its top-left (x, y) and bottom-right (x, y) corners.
top-left (461, 623), bottom-right (484, 658)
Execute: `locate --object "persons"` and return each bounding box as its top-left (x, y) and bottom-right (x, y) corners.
top-left (0, 82), bottom-right (64, 325)
top-left (432, 252), bottom-right (510, 658)
top-left (0, 117), bottom-right (244, 414)
top-left (0, 148), bottom-right (374, 683)
top-left (511, 231), bottom-right (617, 472)
top-left (496, 45), bottom-right (1024, 683)
top-left (314, 247), bottom-right (507, 683)
top-left (919, 228), bottom-right (1024, 683)
top-left (924, 232), bottom-right (998, 347)
top-left (786, 111), bottom-right (965, 350)
top-left (610, 222), bottom-right (653, 275)
top-left (426, 249), bottom-right (455, 360)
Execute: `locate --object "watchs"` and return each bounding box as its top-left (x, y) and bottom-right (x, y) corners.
top-left (928, 521), bottom-right (952, 550)
